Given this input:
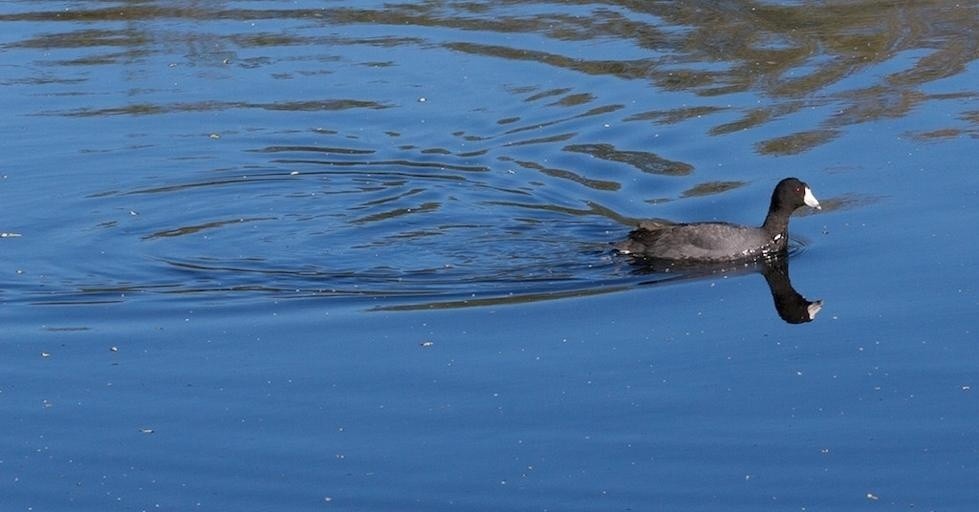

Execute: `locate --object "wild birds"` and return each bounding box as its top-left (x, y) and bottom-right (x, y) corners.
top-left (624, 250), bottom-right (824, 324)
top-left (608, 178), bottom-right (821, 262)
